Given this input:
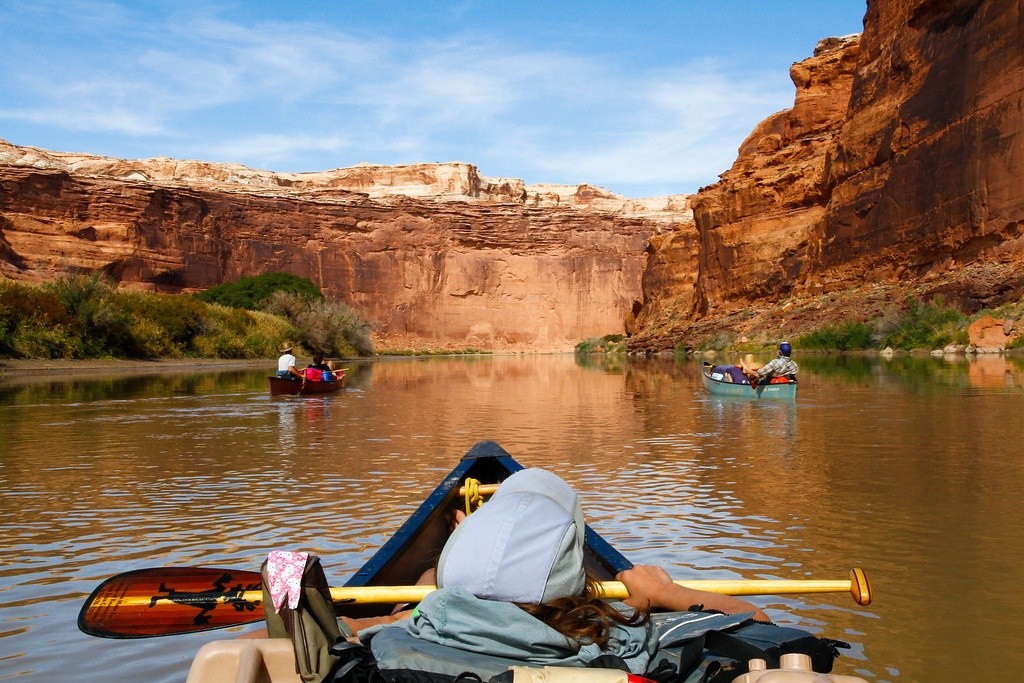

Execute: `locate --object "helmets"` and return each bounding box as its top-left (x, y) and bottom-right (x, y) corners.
top-left (778, 341), bottom-right (791, 357)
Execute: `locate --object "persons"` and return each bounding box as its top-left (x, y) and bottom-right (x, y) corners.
top-left (307, 352), bottom-right (337, 381)
top-left (713, 341), bottom-right (798, 384)
top-left (275, 341), bottom-right (306, 381)
top-left (392, 466), bottom-right (772, 666)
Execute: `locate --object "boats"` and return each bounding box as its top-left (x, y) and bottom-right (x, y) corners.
top-left (696, 356), bottom-right (797, 399)
top-left (235, 437), bottom-right (739, 683)
top-left (268, 371), bottom-right (350, 394)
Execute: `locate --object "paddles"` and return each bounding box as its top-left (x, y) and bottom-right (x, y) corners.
top-left (299, 367), bottom-right (351, 373)
top-left (76, 564), bottom-right (872, 641)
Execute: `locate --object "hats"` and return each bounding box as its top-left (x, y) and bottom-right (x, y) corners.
top-left (436, 468), bottom-right (586, 598)
top-left (278, 341), bottom-right (296, 353)
